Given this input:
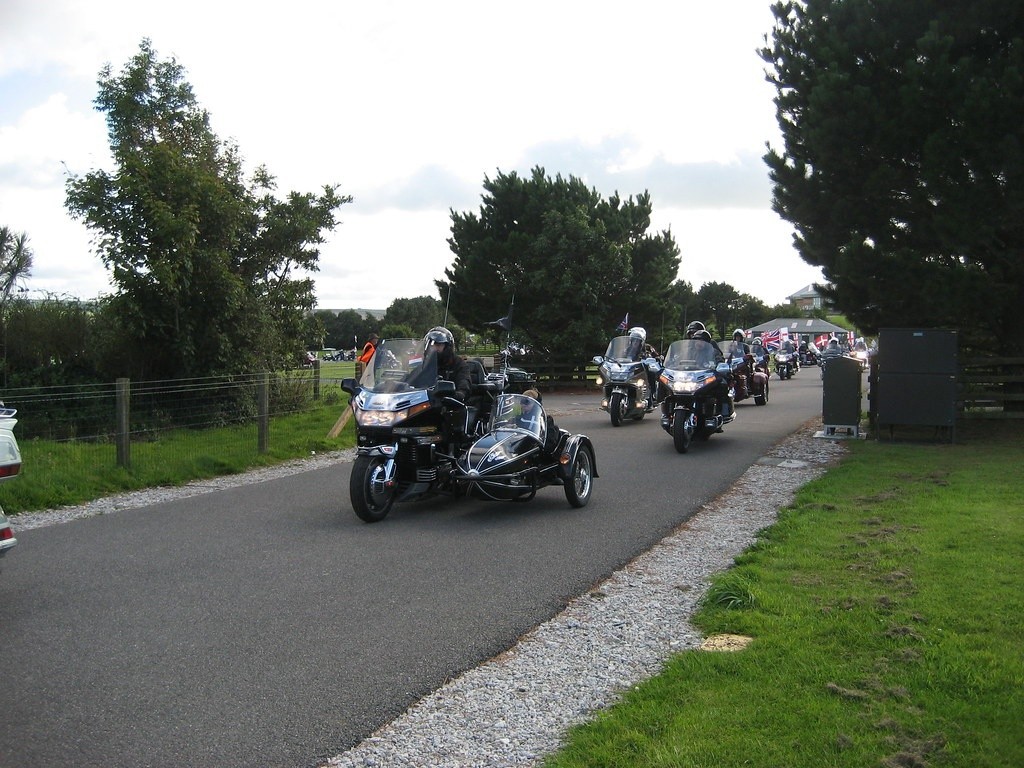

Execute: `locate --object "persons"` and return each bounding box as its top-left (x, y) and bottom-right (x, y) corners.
top-left (325, 333), bottom-right (399, 382)
top-left (502, 389), bottom-right (560, 451)
top-left (397, 327), bottom-right (471, 454)
top-left (603, 321), bottom-right (877, 433)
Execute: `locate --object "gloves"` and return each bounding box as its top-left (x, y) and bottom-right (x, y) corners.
top-left (454, 391), bottom-right (466, 402)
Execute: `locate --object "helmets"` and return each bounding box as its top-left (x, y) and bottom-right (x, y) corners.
top-left (752, 337), bottom-right (764, 346)
top-left (732, 329), bottom-right (746, 341)
top-left (829, 337), bottom-right (839, 344)
top-left (686, 321), bottom-right (705, 334)
top-left (628, 327), bottom-right (646, 346)
top-left (424, 326), bottom-right (455, 355)
top-left (692, 330), bottom-right (711, 343)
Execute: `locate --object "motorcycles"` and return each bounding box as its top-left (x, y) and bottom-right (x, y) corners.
top-left (322, 348), bottom-right (336, 361)
top-left (798, 345), bottom-right (821, 367)
top-left (717, 341), bottom-right (769, 405)
top-left (340, 339), bottom-right (600, 523)
top-left (748, 345), bottom-right (771, 379)
top-left (303, 352), bottom-right (316, 368)
top-left (850, 342), bottom-right (868, 370)
top-left (648, 340), bottom-right (737, 454)
top-left (816, 344), bottom-right (849, 380)
top-left (771, 342), bottom-right (797, 380)
top-left (590, 336), bottom-right (664, 426)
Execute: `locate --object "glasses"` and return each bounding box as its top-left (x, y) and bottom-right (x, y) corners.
top-left (520, 399), bottom-right (532, 405)
top-left (631, 338), bottom-right (641, 343)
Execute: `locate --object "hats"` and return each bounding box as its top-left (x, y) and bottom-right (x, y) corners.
top-left (369, 333), bottom-right (380, 340)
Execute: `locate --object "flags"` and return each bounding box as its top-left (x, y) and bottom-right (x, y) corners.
top-left (813, 332), bottom-right (830, 349)
top-left (761, 327), bottom-right (789, 352)
top-left (482, 303), bottom-right (514, 332)
top-left (616, 313), bottom-right (629, 332)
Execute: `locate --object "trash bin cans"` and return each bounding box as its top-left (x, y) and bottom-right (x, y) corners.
top-left (823, 355), bottom-right (863, 437)
